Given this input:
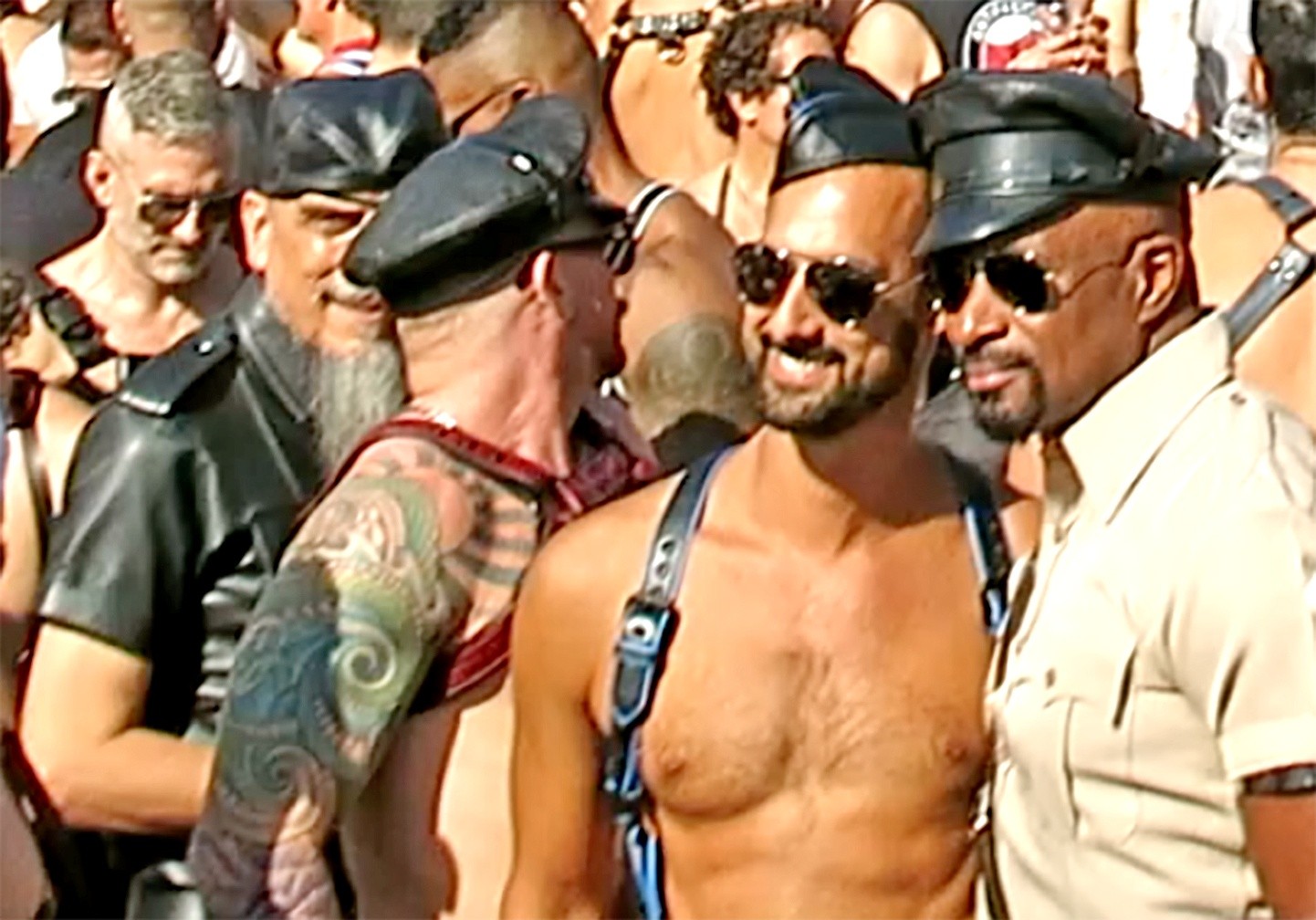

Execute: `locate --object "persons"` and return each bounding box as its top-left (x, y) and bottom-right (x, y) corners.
top-left (0, 0), bottom-right (1316, 920)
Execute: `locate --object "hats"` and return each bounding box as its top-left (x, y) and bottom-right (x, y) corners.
top-left (904, 69), bottom-right (1208, 264)
top-left (250, 69), bottom-right (449, 200)
top-left (770, 56), bottom-right (925, 192)
top-left (340, 97), bottom-right (627, 317)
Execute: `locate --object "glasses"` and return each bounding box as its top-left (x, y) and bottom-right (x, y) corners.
top-left (924, 249), bottom-right (1130, 316)
top-left (725, 241), bottom-right (924, 326)
top-left (105, 153), bottom-right (243, 230)
top-left (516, 224), bottom-right (635, 292)
top-left (8, 368), bottom-right (45, 428)
